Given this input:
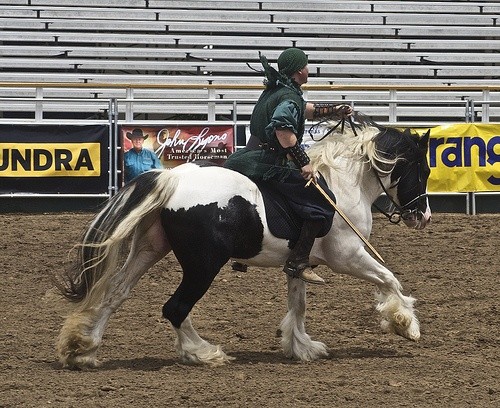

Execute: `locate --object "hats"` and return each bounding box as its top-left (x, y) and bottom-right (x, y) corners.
top-left (126, 128), bottom-right (149, 140)
top-left (277, 48), bottom-right (309, 76)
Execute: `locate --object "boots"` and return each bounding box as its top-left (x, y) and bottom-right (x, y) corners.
top-left (231, 262), bottom-right (249, 272)
top-left (282, 220), bottom-right (327, 285)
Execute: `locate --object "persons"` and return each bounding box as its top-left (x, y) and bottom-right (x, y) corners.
top-left (124, 128), bottom-right (162, 179)
top-left (231, 48), bottom-right (354, 284)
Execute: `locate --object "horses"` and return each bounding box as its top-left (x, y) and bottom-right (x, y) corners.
top-left (50, 125), bottom-right (430, 369)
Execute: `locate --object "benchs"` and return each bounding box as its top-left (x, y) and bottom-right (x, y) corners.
top-left (0, 0), bottom-right (500, 124)
